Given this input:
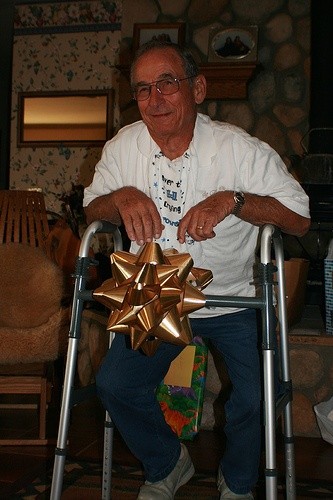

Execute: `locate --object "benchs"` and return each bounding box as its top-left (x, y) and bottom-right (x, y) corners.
top-left (76, 307), bottom-right (333, 437)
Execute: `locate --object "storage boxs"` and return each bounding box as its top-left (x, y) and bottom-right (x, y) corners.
top-left (156, 346), bottom-right (208, 439)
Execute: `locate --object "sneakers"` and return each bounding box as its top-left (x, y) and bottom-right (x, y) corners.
top-left (138, 443), bottom-right (195, 500)
top-left (217, 468), bottom-right (253, 500)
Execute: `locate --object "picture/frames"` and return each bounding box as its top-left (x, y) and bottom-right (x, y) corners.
top-left (207, 25), bottom-right (257, 62)
top-left (132, 22), bottom-right (185, 57)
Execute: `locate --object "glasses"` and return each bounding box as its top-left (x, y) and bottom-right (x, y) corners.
top-left (133, 75), bottom-right (194, 102)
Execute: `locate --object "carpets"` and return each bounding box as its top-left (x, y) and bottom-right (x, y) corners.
top-left (0, 456), bottom-right (333, 500)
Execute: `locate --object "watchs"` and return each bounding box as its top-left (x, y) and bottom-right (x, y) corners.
top-left (233, 191), bottom-right (244, 218)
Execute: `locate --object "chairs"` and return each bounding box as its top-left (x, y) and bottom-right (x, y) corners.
top-left (0, 190), bottom-right (63, 445)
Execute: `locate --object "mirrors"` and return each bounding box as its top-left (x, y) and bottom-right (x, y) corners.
top-left (16, 89), bottom-right (115, 146)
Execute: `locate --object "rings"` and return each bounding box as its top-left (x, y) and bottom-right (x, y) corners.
top-left (195, 224), bottom-right (203, 231)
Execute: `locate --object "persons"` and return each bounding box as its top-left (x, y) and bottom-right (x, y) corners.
top-left (80, 45), bottom-right (312, 499)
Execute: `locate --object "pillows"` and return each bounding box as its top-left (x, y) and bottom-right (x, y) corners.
top-left (0, 243), bottom-right (64, 328)
